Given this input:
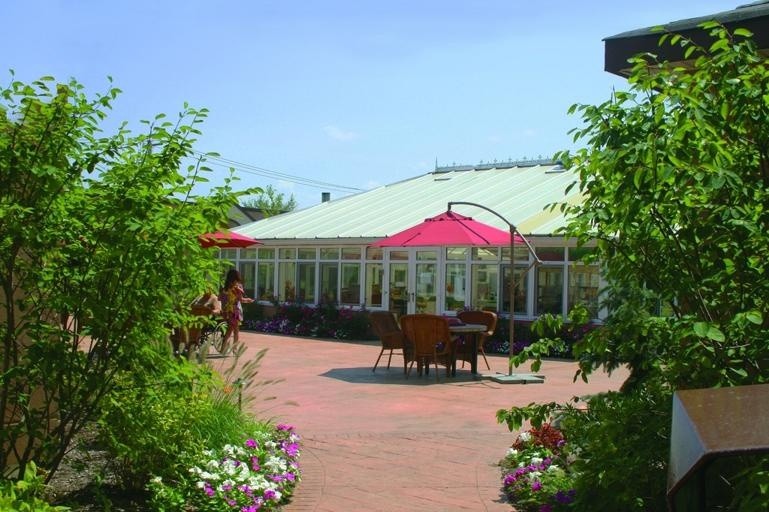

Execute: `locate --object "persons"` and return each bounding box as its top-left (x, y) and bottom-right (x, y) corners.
top-left (217, 268), bottom-right (255, 356)
top-left (172, 285), bottom-right (223, 358)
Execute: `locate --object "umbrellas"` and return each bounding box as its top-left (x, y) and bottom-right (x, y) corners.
top-left (196, 228), bottom-right (265, 255)
top-left (365, 199), bottom-right (544, 378)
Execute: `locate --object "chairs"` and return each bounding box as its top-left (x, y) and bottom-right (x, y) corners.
top-left (368, 310), bottom-right (497, 383)
